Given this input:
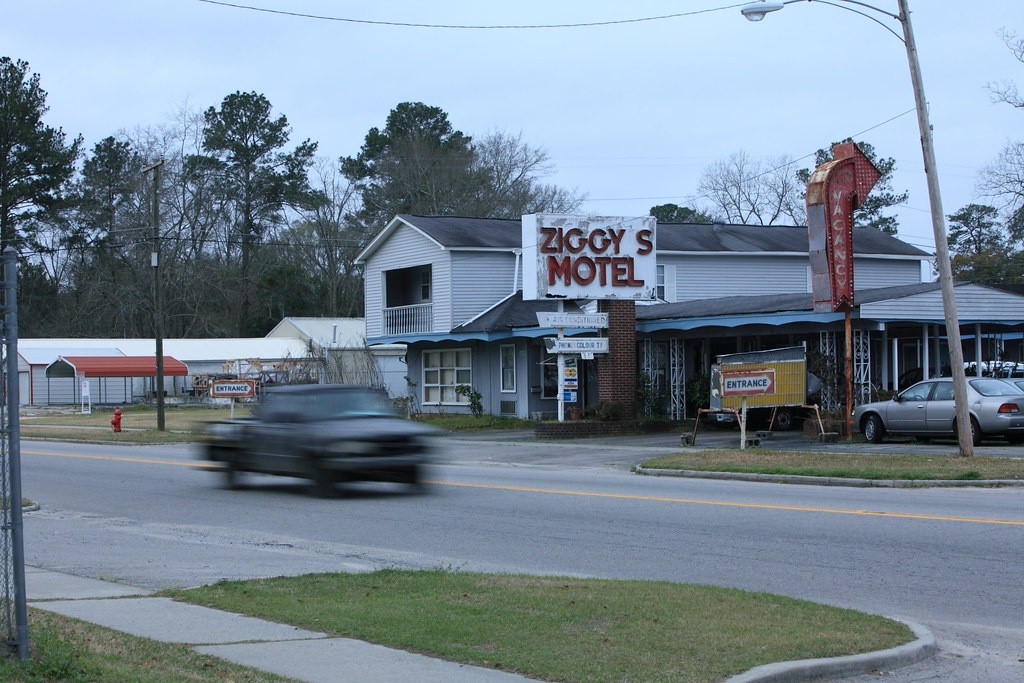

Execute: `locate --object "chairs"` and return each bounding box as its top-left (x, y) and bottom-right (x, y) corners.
top-left (937, 392), bottom-right (952, 400)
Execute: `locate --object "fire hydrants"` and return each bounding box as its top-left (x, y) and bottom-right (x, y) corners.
top-left (111, 407), bottom-right (122, 432)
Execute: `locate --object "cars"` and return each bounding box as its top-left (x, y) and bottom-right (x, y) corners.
top-left (964, 361), bottom-right (1024, 375)
top-left (999, 378), bottom-right (1024, 391)
top-left (853, 377), bottom-right (1024, 444)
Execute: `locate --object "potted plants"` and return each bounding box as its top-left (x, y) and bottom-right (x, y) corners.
top-left (544, 365), bottom-right (587, 420)
top-left (634, 371), bottom-right (671, 431)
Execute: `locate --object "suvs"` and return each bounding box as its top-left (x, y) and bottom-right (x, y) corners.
top-left (706, 356), bottom-right (820, 431)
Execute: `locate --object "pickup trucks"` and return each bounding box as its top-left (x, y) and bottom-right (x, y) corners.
top-left (197, 384), bottom-right (445, 497)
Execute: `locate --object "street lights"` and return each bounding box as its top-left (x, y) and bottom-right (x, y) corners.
top-left (740, 0), bottom-right (976, 456)
top-left (105, 240), bottom-right (165, 430)
